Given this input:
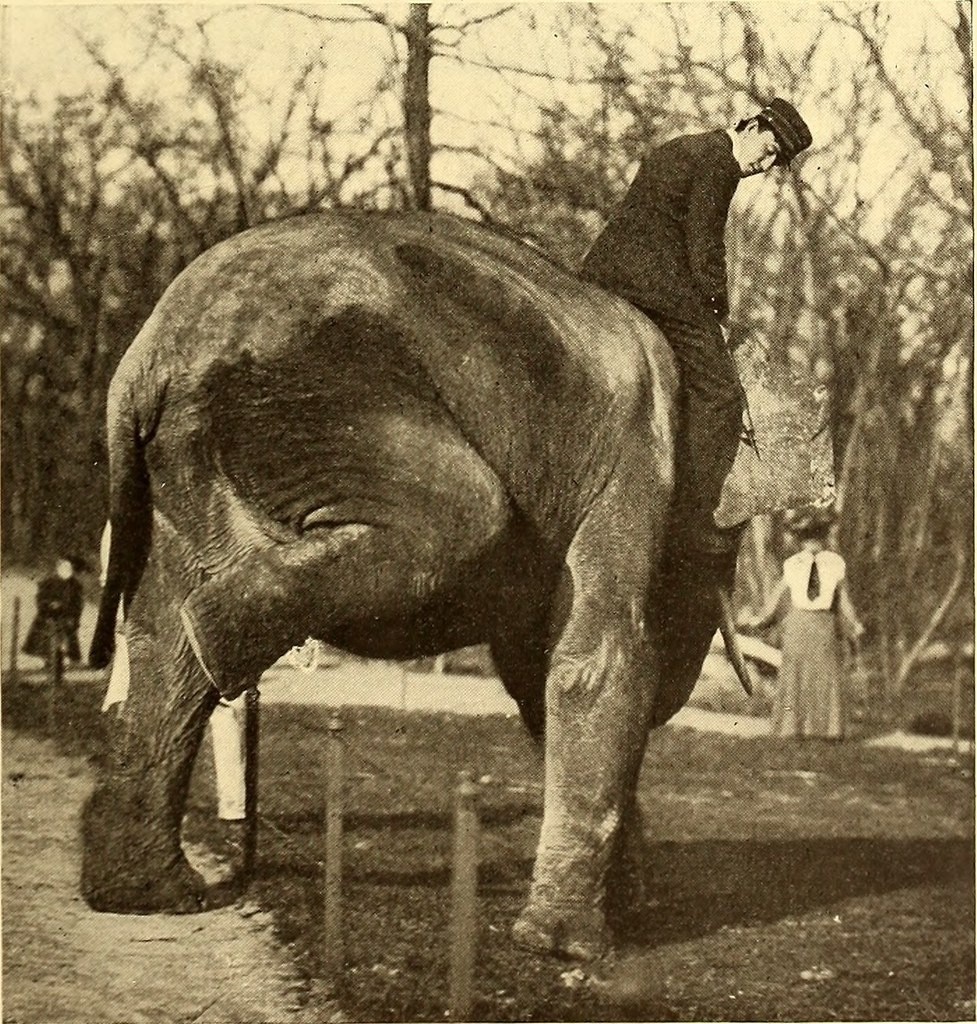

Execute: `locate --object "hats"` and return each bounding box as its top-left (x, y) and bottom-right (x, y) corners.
top-left (784, 505), bottom-right (836, 533)
top-left (758, 97), bottom-right (812, 172)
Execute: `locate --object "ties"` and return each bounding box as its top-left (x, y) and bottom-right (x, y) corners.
top-left (807, 558), bottom-right (821, 602)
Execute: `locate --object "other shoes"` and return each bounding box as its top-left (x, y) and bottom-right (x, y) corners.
top-left (667, 518), bottom-right (736, 556)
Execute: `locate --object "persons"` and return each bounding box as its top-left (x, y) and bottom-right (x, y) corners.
top-left (587, 98), bottom-right (811, 554)
top-left (100, 516), bottom-right (247, 831)
top-left (19, 559), bottom-right (82, 674)
top-left (782, 525), bottom-right (865, 740)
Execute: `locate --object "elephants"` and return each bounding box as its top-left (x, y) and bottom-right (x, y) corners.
top-left (83, 204), bottom-right (753, 959)
